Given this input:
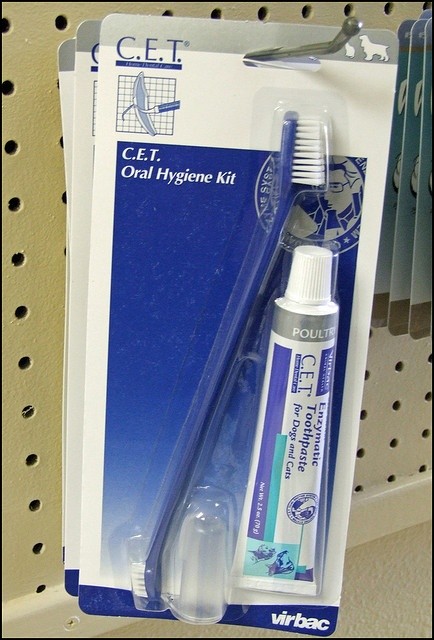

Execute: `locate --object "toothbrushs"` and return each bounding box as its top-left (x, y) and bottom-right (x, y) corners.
top-left (131, 112), bottom-right (329, 602)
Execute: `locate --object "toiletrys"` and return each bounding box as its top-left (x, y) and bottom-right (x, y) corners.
top-left (228, 245), bottom-right (339, 598)
